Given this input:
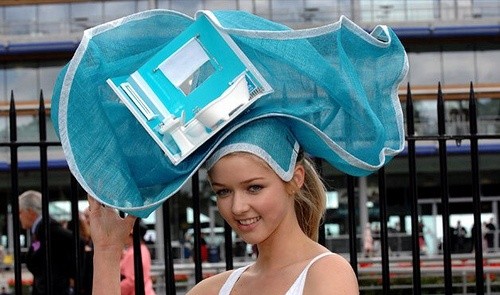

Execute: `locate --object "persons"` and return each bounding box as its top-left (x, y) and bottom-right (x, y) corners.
top-left (361, 222), bottom-right (374, 258)
top-left (18, 190), bottom-right (71, 295)
top-left (88, 119), bottom-right (359, 295)
top-left (65, 206), bottom-right (332, 295)
top-left (396, 221), bottom-right (496, 252)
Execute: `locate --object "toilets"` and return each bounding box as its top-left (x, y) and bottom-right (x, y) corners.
top-left (181, 108), bottom-right (210, 146)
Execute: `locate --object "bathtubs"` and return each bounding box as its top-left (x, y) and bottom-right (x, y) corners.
top-left (195, 74), bottom-right (249, 130)
